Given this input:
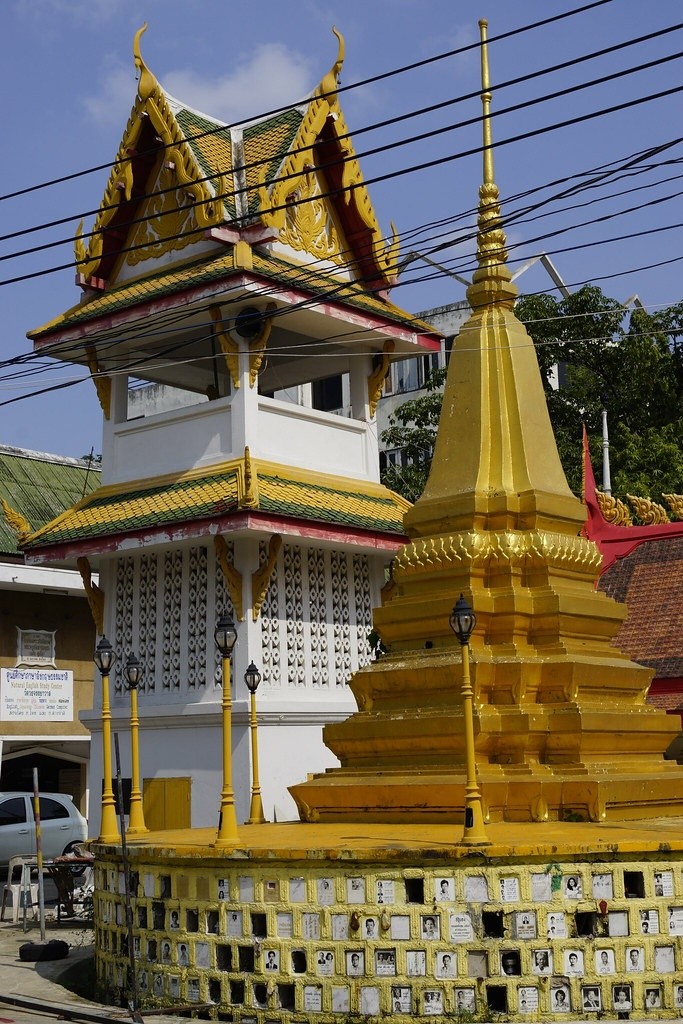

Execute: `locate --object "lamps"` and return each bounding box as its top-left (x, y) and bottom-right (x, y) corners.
top-left (122, 651), bottom-right (151, 834)
top-left (214, 603), bottom-right (243, 846)
top-left (91, 633), bottom-right (123, 845)
top-left (244, 660), bottom-right (271, 825)
top-left (447, 592), bottom-right (495, 848)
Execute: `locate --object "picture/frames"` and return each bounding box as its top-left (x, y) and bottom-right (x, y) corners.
top-left (13, 625), bottom-right (58, 669)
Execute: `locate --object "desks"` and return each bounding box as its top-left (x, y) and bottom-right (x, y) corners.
top-left (25, 862), bottom-right (95, 926)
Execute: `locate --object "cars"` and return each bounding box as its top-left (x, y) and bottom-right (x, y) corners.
top-left (0, 792), bottom-right (89, 882)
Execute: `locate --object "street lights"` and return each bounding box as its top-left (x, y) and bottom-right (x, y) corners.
top-left (214, 609), bottom-right (244, 846)
top-left (123, 650), bottom-right (150, 835)
top-left (245, 660), bottom-right (269, 825)
top-left (92, 633), bottom-right (123, 843)
top-left (450, 594), bottom-right (490, 845)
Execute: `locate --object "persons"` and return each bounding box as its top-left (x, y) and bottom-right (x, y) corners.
top-left (140, 973), bottom-right (147, 988)
top-left (188, 979), bottom-right (199, 990)
top-left (219, 892), bottom-right (224, 898)
top-left (319, 952), bottom-right (333, 964)
top-left (232, 915), bottom-right (237, 921)
top-left (567, 878), bottom-right (581, 898)
top-left (157, 976), bottom-right (162, 986)
top-left (324, 881), bottom-right (384, 903)
top-left (441, 955), bottom-right (453, 973)
top-left (378, 953), bottom-right (394, 965)
top-left (366, 919), bottom-right (375, 936)
top-left (440, 880), bottom-right (450, 900)
top-left (500, 879), bottom-right (506, 900)
top-left (135, 938), bottom-right (140, 951)
top-left (266, 951), bottom-right (277, 969)
top-left (654, 874), bottom-right (664, 896)
top-left (171, 911), bottom-right (179, 928)
top-left (392, 988), bottom-right (465, 1013)
top-left (163, 943), bottom-right (187, 961)
top-left (502, 908), bottom-right (683, 1012)
top-left (351, 954), bottom-right (359, 968)
top-left (423, 917), bottom-right (435, 936)
top-left (219, 880), bottom-right (224, 886)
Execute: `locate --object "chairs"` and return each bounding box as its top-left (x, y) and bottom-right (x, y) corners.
top-left (1, 854), bottom-right (40, 923)
top-left (61, 839), bottom-right (87, 888)
